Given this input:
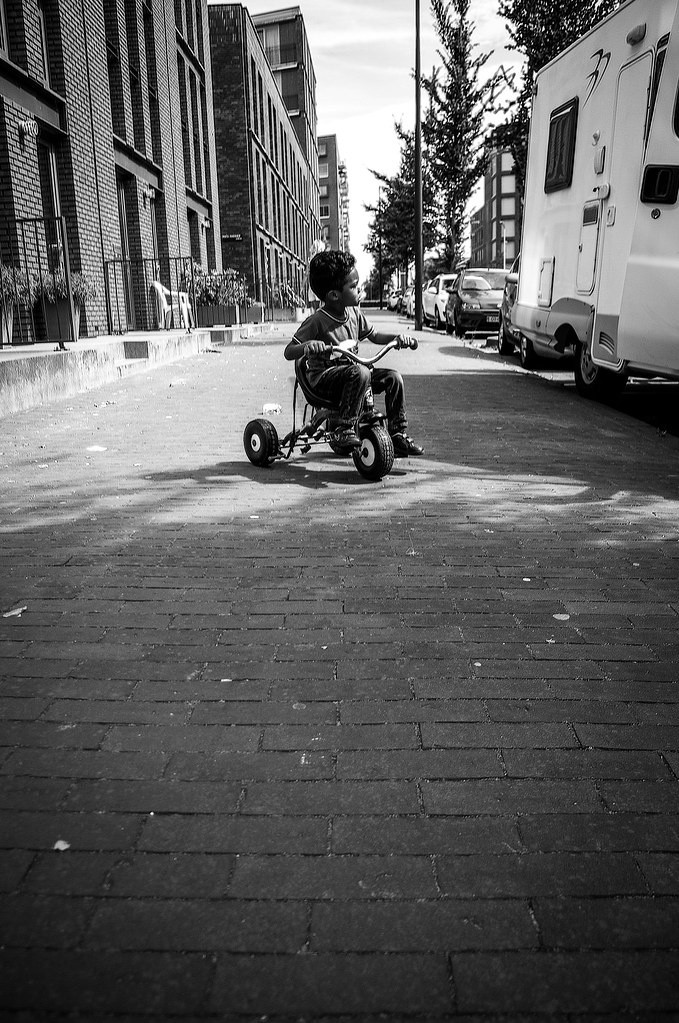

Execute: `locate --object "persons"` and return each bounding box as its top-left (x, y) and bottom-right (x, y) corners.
top-left (284, 250), bottom-right (425, 455)
top-left (300, 240), bottom-right (326, 314)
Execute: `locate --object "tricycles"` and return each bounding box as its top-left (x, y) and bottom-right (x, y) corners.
top-left (243, 335), bottom-right (419, 481)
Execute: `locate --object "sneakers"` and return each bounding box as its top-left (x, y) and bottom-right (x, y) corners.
top-left (333, 424), bottom-right (362, 446)
top-left (392, 430), bottom-right (424, 456)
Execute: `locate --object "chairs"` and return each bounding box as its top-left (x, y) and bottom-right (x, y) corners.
top-left (151, 281), bottom-right (195, 329)
top-left (464, 280), bottom-right (477, 288)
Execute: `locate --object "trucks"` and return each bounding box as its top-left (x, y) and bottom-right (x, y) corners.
top-left (515, 0), bottom-right (679, 410)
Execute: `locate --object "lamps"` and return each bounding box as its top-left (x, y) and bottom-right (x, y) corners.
top-left (201, 220), bottom-right (210, 228)
top-left (143, 189), bottom-right (155, 198)
top-left (18, 120), bottom-right (38, 136)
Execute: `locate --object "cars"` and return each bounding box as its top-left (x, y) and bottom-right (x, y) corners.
top-left (423, 273), bottom-right (493, 330)
top-left (444, 267), bottom-right (511, 338)
top-left (386, 284), bottom-right (426, 319)
top-left (498, 252), bottom-right (545, 370)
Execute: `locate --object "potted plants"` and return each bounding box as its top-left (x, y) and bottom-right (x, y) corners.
top-left (0, 264), bottom-right (95, 349)
top-left (180, 261), bottom-right (266, 327)
top-left (265, 282), bottom-right (315, 321)
